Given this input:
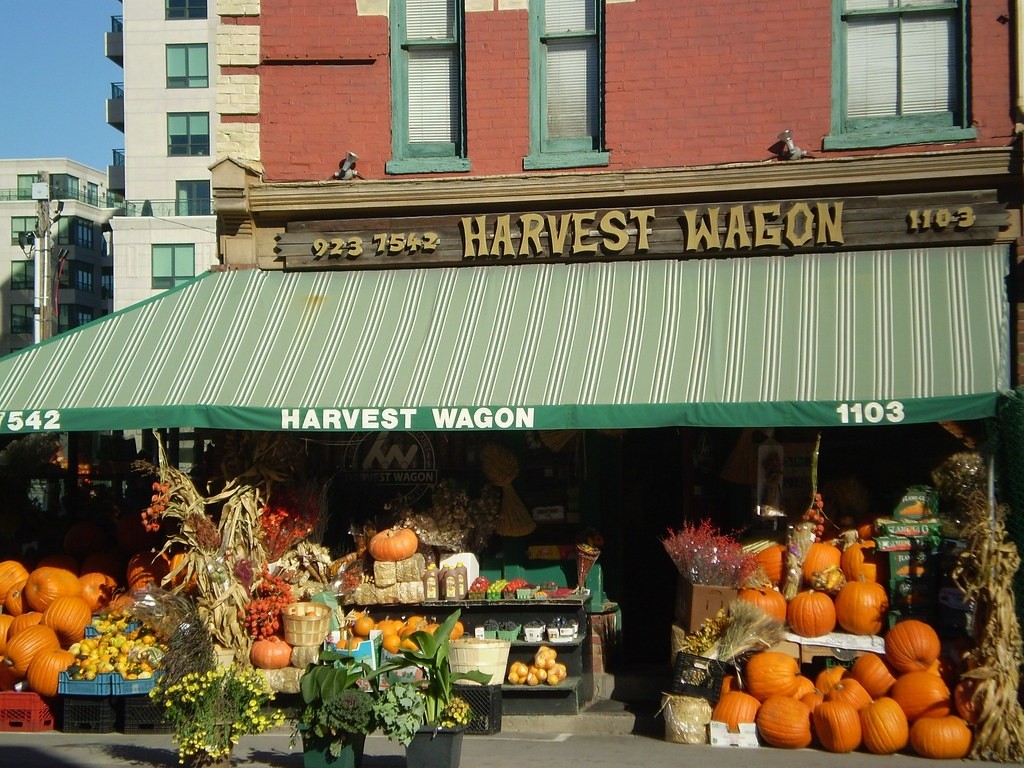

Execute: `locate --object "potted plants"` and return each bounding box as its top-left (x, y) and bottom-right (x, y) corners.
top-left (288, 652), bottom-right (382, 768)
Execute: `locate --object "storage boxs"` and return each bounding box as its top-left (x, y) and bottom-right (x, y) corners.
top-left (870, 514), bottom-right (943, 628)
top-left (0, 586), bottom-right (577, 768)
top-left (671, 652), bottom-right (731, 701)
top-left (674, 581), bottom-right (737, 633)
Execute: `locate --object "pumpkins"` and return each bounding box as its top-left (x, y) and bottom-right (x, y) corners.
top-left (0, 526), bottom-right (981, 756)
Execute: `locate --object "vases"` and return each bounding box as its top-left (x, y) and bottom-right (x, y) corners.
top-left (406, 724), bottom-right (468, 768)
top-left (191, 722), bottom-right (234, 768)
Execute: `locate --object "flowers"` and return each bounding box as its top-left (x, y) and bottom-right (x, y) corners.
top-left (148, 664), bottom-right (286, 763)
top-left (361, 609), bottom-right (472, 747)
top-left (659, 518), bottom-right (766, 588)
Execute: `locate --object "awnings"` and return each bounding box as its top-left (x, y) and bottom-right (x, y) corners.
top-left (0, 243), bottom-right (1009, 432)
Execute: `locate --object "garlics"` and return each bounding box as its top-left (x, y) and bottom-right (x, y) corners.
top-left (384, 482), bottom-right (501, 546)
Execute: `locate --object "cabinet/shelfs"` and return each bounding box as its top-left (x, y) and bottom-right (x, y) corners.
top-left (336, 584), bottom-right (589, 712)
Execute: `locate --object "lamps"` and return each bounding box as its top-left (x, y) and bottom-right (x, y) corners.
top-left (335, 151), bottom-right (358, 180)
top-left (777, 130), bottom-right (805, 162)
